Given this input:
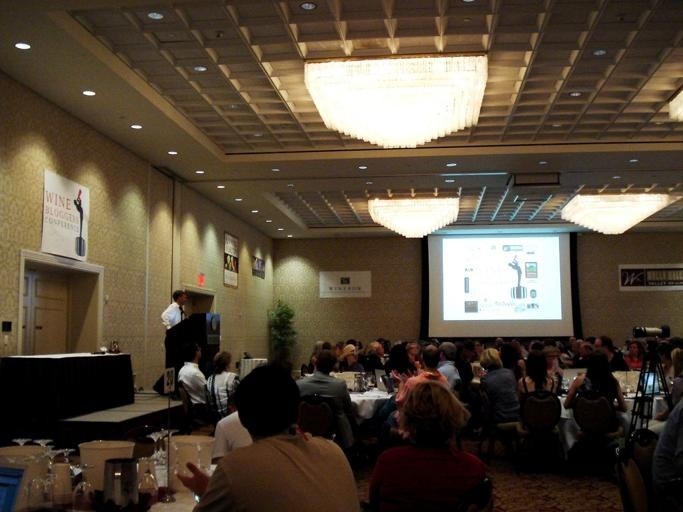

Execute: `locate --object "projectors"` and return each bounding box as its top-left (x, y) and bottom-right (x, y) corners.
top-left (506, 172), bottom-right (561, 195)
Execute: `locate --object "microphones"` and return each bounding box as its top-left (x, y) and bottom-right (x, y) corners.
top-left (179, 306), bottom-right (188, 317)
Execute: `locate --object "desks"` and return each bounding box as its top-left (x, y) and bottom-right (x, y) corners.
top-left (0, 353), bottom-right (135, 419)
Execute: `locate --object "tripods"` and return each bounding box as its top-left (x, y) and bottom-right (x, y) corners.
top-left (630, 341), bottom-right (675, 434)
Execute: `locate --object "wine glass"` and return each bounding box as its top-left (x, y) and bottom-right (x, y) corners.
top-left (135, 457), bottom-right (159, 504)
top-left (171, 441), bottom-right (209, 502)
top-left (146, 428), bottom-right (179, 467)
top-left (8, 431), bottom-right (97, 512)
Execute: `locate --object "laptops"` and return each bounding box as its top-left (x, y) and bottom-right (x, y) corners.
top-left (641, 371), bottom-right (672, 397)
top-left (381, 375), bottom-right (398, 396)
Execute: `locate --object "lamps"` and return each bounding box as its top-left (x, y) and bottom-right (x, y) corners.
top-left (668, 87), bottom-right (683, 122)
top-left (369, 197), bottom-right (459, 242)
top-left (559, 191), bottom-right (681, 236)
top-left (305, 54), bottom-right (490, 150)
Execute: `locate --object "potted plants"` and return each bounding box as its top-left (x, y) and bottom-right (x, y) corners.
top-left (267, 296), bottom-right (297, 370)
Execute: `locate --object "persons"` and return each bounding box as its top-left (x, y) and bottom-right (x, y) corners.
top-left (175, 361), bottom-right (361, 512)
top-left (295, 323), bottom-right (682, 475)
top-left (649, 400), bottom-right (682, 511)
top-left (176, 342), bottom-right (207, 426)
top-left (204, 351), bottom-right (240, 418)
top-left (151, 290), bottom-right (186, 395)
top-left (209, 392), bottom-right (255, 467)
top-left (367, 375), bottom-right (488, 511)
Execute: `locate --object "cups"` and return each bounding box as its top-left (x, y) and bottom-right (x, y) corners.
top-left (102, 456), bottom-right (139, 512)
